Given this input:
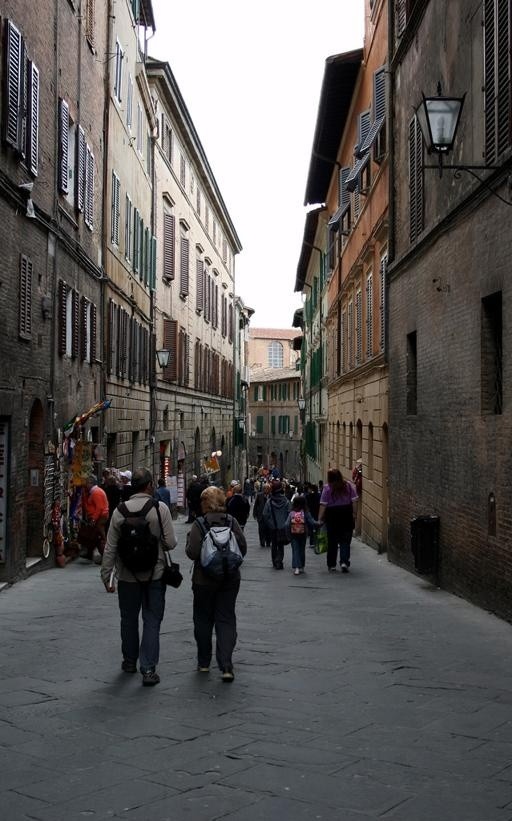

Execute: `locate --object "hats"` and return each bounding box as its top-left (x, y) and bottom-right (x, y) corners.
top-left (292, 496), bottom-right (306, 506)
top-left (120, 469), bottom-right (132, 481)
top-left (271, 479), bottom-right (283, 491)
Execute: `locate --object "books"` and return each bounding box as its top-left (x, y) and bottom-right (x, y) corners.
top-left (110, 572), bottom-right (114, 588)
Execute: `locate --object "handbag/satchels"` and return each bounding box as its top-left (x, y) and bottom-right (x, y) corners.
top-left (274, 528), bottom-right (291, 544)
top-left (161, 563), bottom-right (183, 588)
top-left (76, 523), bottom-right (102, 550)
top-left (313, 526), bottom-right (328, 554)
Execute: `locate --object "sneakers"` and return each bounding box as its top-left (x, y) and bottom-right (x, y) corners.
top-left (299, 567), bottom-right (305, 572)
top-left (143, 672), bottom-right (160, 686)
top-left (221, 666), bottom-right (234, 680)
top-left (341, 563), bottom-right (350, 573)
top-left (294, 567), bottom-right (299, 574)
top-left (120, 658), bottom-right (137, 673)
top-left (197, 664), bottom-right (209, 672)
top-left (328, 566), bottom-right (336, 571)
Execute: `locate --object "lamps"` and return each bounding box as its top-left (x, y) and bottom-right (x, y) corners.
top-left (239, 420), bottom-right (245, 429)
top-left (297, 399), bottom-right (307, 412)
top-left (411, 81), bottom-right (500, 179)
top-left (156, 349), bottom-right (171, 374)
top-left (289, 431), bottom-right (294, 440)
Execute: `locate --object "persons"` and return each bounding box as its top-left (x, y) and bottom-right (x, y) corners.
top-left (185, 486), bottom-right (245, 683)
top-left (79, 464), bottom-right (362, 575)
top-left (100, 469), bottom-right (177, 686)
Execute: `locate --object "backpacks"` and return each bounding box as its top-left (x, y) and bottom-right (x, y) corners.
top-left (290, 510), bottom-right (306, 537)
top-left (195, 512), bottom-right (245, 583)
top-left (116, 497), bottom-right (162, 572)
